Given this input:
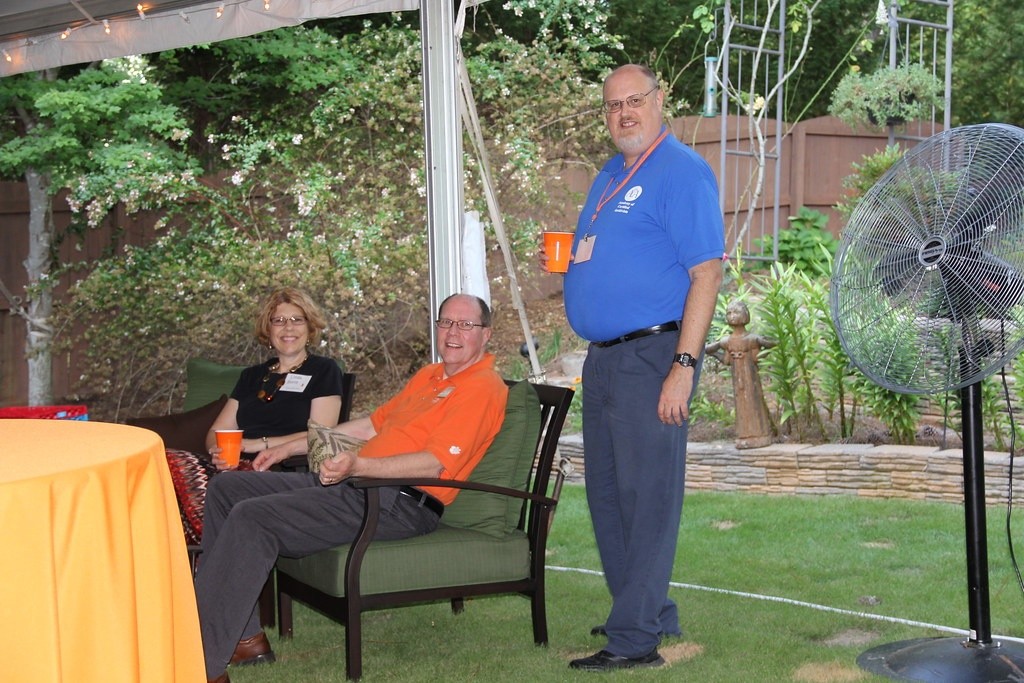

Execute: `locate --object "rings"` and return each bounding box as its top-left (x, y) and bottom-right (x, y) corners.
top-left (329, 478), bottom-right (333, 483)
top-left (208, 449), bottom-right (211, 454)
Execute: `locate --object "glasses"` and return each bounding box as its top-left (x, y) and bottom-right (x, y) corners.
top-left (603, 85), bottom-right (659, 113)
top-left (434, 319), bottom-right (483, 332)
top-left (270, 317), bottom-right (307, 326)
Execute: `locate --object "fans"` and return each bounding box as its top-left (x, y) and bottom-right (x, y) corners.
top-left (829, 123), bottom-right (1024, 683)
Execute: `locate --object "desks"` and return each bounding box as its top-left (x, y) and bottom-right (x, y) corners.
top-left (0, 418), bottom-right (209, 683)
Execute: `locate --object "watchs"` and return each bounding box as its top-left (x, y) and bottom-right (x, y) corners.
top-left (673, 352), bottom-right (696, 367)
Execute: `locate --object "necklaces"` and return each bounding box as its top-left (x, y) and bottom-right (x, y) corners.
top-left (257, 352), bottom-right (309, 402)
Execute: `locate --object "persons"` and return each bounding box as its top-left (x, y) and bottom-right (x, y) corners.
top-left (538, 63), bottom-right (725, 670)
top-left (192, 294), bottom-right (512, 683)
top-left (165, 291), bottom-right (343, 568)
top-left (705, 300), bottom-right (777, 449)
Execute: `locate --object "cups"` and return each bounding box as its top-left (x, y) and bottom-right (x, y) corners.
top-left (215, 429), bottom-right (244, 467)
top-left (542, 231), bottom-right (575, 273)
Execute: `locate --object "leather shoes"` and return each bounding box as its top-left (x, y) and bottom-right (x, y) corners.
top-left (230, 631), bottom-right (276, 668)
top-left (570, 647), bottom-right (666, 670)
top-left (590, 626), bottom-right (608, 638)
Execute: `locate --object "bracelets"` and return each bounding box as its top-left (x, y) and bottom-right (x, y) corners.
top-left (261, 437), bottom-right (269, 449)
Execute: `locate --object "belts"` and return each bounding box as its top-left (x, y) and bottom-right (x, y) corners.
top-left (593, 321), bottom-right (679, 347)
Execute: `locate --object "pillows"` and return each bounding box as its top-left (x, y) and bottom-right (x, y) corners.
top-left (123, 393), bottom-right (232, 469)
top-left (186, 360), bottom-right (347, 414)
top-left (437, 378), bottom-right (545, 542)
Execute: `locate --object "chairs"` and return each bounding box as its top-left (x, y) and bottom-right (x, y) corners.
top-left (281, 379), bottom-right (576, 683)
top-left (152, 360), bottom-right (358, 621)
